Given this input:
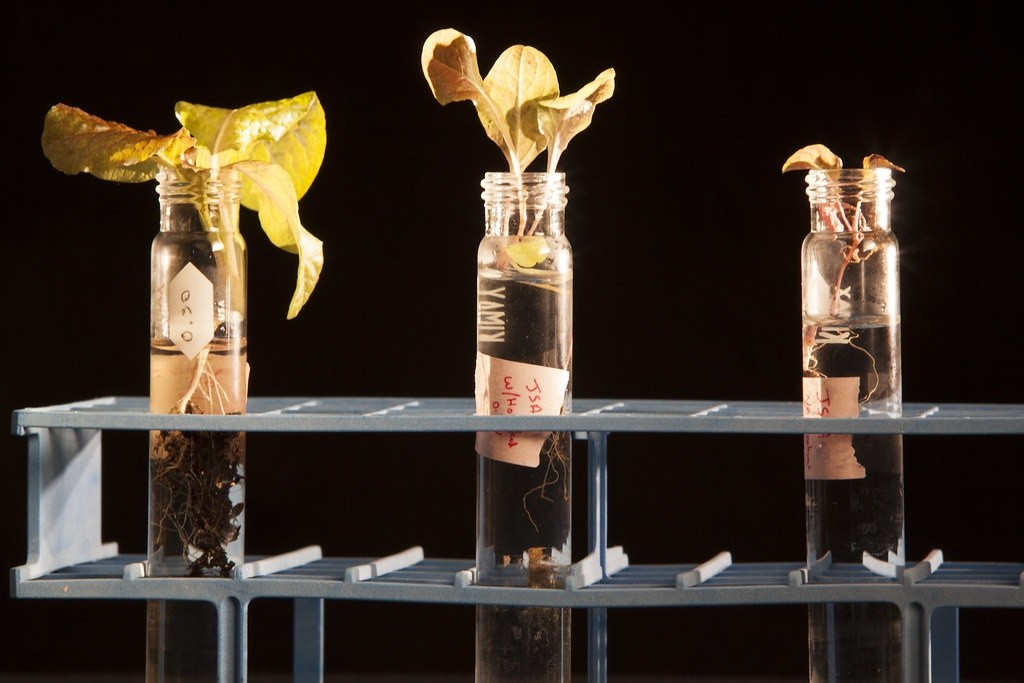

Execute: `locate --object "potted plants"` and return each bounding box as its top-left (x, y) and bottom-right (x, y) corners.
top-left (422, 26), bottom-right (616, 683)
top-left (42, 90), bottom-right (325, 683)
top-left (784, 142), bottom-right (906, 681)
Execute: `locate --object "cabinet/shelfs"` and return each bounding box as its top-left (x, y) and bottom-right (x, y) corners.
top-left (10, 396), bottom-right (1024, 683)
